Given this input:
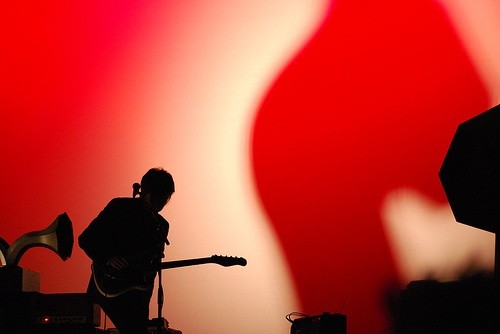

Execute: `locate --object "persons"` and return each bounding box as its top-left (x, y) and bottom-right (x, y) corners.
top-left (76, 168), bottom-right (174, 333)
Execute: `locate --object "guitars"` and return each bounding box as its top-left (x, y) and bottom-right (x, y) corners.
top-left (91, 253), bottom-right (247, 298)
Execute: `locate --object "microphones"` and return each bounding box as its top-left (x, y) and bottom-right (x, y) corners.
top-left (132, 183), bottom-right (141, 198)
